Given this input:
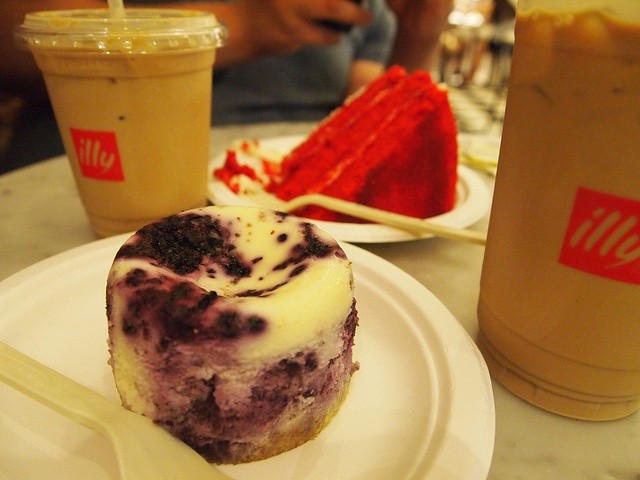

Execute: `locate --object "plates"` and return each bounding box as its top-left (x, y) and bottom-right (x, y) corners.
top-left (208, 135), bottom-right (493, 243)
top-left (0, 230), bottom-right (496, 479)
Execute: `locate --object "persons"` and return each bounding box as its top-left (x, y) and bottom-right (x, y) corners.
top-left (2, 0), bottom-right (370, 78)
top-left (212, 1), bottom-right (396, 123)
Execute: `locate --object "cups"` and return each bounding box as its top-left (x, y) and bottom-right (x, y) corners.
top-left (477, 0), bottom-right (640, 421)
top-left (23, 9), bottom-right (227, 237)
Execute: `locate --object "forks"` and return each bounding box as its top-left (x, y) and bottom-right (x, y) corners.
top-left (248, 191), bottom-right (489, 240)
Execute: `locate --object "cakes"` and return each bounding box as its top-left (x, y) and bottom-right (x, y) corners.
top-left (104, 206), bottom-right (359, 466)
top-left (277, 65), bottom-right (459, 221)
